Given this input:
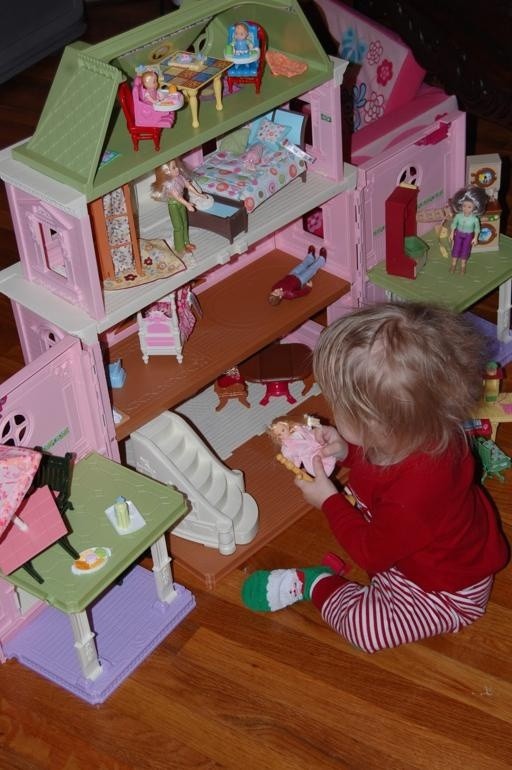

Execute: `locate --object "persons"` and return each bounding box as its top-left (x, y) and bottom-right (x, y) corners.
top-left (448, 187), bottom-right (489, 275)
top-left (151, 159), bottom-right (209, 256)
top-left (240, 302), bottom-right (511, 652)
top-left (138, 71), bottom-right (164, 103)
top-left (265, 418), bottom-right (340, 479)
top-left (231, 23), bottom-right (254, 69)
top-left (268, 245), bottom-right (327, 305)
